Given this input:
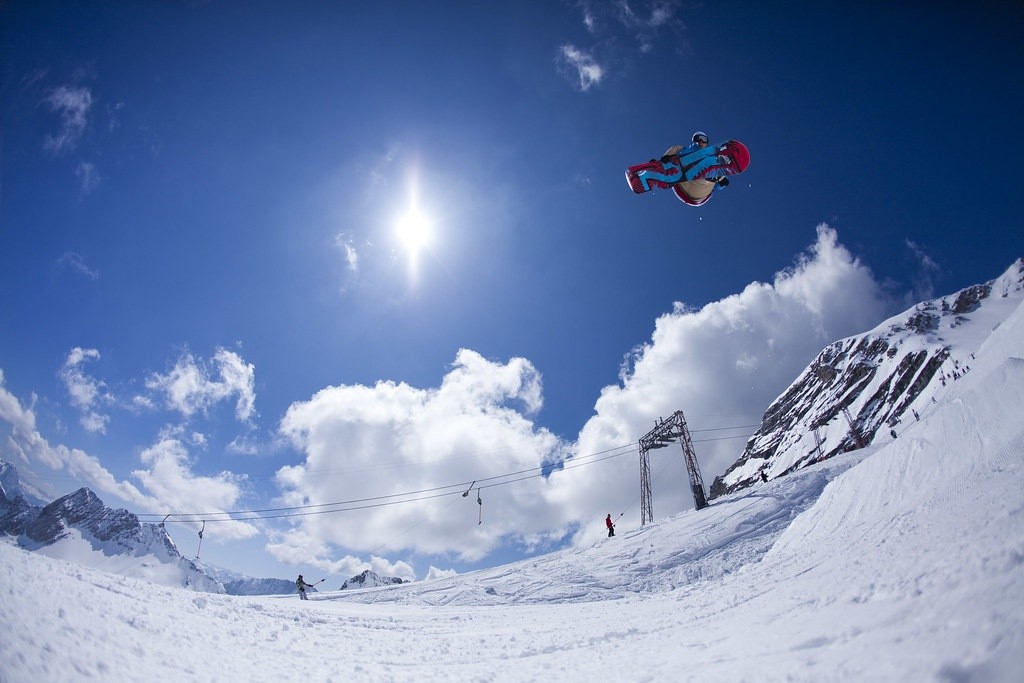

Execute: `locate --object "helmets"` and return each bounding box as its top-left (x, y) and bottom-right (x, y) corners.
top-left (691, 131), bottom-right (709, 145)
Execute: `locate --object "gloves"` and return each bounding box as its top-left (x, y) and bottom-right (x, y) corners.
top-left (301, 587), bottom-right (305, 591)
top-left (309, 584), bottom-right (313, 588)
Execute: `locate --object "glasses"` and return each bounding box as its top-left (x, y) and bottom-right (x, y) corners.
top-left (693, 134), bottom-right (708, 143)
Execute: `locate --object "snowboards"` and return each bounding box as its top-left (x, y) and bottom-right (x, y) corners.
top-left (623, 138), bottom-right (751, 195)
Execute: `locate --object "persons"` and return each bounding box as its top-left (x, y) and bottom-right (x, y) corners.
top-left (648, 131), bottom-right (730, 207)
top-left (296, 575), bottom-right (313, 600)
top-left (605, 514), bottom-right (616, 537)
